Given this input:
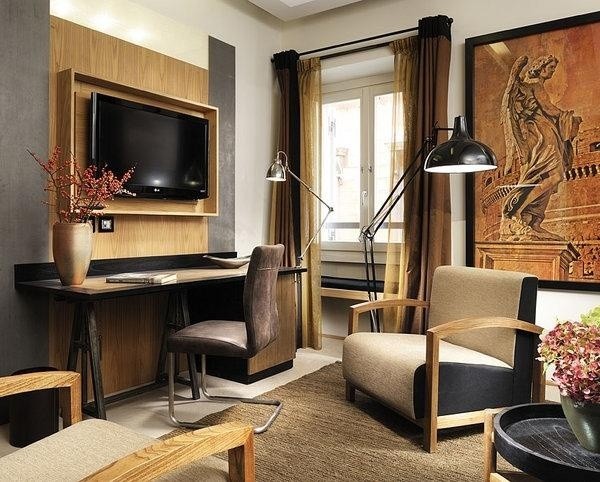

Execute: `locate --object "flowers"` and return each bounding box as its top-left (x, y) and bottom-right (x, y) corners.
top-left (26, 147), bottom-right (138, 223)
top-left (535, 306), bottom-right (600, 407)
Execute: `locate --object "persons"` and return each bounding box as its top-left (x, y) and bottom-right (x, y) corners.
top-left (501, 50), bottom-right (583, 244)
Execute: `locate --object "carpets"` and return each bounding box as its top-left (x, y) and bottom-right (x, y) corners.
top-left (157, 358), bottom-right (561, 482)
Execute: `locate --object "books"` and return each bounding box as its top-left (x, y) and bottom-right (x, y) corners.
top-left (106, 272), bottom-right (179, 285)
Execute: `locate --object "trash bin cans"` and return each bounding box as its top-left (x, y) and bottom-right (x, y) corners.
top-left (9, 366), bottom-right (59, 447)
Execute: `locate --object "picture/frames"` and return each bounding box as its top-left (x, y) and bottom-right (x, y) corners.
top-left (465, 11), bottom-right (600, 291)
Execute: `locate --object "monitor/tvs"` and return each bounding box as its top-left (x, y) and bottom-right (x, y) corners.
top-left (88, 91), bottom-right (209, 200)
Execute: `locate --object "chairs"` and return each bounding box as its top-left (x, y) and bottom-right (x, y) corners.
top-left (342, 265), bottom-right (544, 453)
top-left (0, 370), bottom-right (255, 482)
top-left (166, 243), bottom-right (285, 435)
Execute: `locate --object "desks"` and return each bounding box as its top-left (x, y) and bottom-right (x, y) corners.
top-left (14, 252), bottom-right (309, 422)
top-left (483, 402), bottom-right (600, 482)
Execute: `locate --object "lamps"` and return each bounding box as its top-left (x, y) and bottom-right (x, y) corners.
top-left (265, 151), bottom-right (335, 267)
top-left (359, 116), bottom-right (497, 332)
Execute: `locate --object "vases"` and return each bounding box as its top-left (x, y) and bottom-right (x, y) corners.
top-left (560, 395), bottom-right (600, 452)
top-left (53, 225), bottom-right (93, 284)
